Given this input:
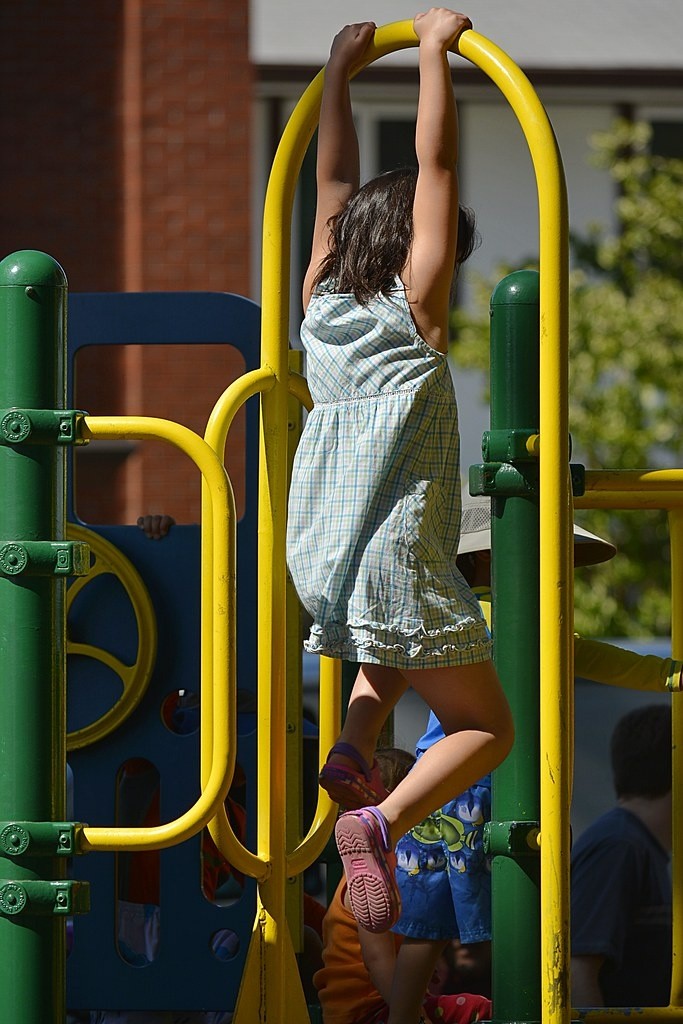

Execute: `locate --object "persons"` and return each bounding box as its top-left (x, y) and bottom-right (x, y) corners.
top-left (91, 483), bottom-right (616, 1024)
top-left (287, 8), bottom-right (514, 932)
top-left (570, 704), bottom-right (672, 1009)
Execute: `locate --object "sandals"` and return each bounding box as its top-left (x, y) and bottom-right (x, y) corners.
top-left (335, 805), bottom-right (402, 934)
top-left (317, 741), bottom-right (390, 811)
